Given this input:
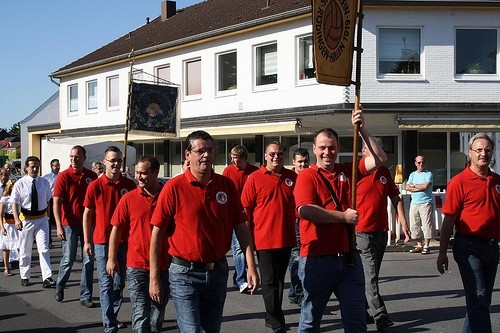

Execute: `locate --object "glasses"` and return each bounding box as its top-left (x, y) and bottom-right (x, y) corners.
top-left (269, 152), bottom-right (284, 157)
top-left (98, 167), bottom-right (103, 170)
top-left (418, 162), bottom-right (424, 165)
top-left (106, 159), bottom-right (122, 164)
top-left (471, 148), bottom-right (492, 155)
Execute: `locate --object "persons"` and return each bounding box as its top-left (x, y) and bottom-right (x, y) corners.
top-left (406, 156), bottom-right (435, 254)
top-left (182, 158), bottom-right (190, 173)
top-left (436, 133), bottom-right (499, 333)
top-left (293, 103), bottom-right (386, 333)
top-left (44, 159), bottom-right (62, 248)
top-left (355, 137), bottom-right (412, 331)
top-left (83, 145), bottom-right (137, 333)
top-left (9, 156), bottom-right (57, 288)
top-left (105, 155), bottom-right (174, 333)
top-left (52, 145), bottom-right (98, 308)
top-left (240, 141), bottom-right (299, 333)
top-left (149, 131), bottom-right (260, 333)
top-left (223, 146), bottom-right (260, 293)
top-left (289, 148), bottom-right (313, 307)
top-left (91, 161), bottom-right (104, 176)
top-left (0, 163), bottom-right (22, 277)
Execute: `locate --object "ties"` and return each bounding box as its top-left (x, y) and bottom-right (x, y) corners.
top-left (31, 181), bottom-right (39, 214)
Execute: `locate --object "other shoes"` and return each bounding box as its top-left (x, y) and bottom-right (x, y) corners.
top-left (409, 247), bottom-right (421, 253)
top-left (56, 287), bottom-right (64, 301)
top-left (116, 319), bottom-right (126, 328)
top-left (43, 278), bottom-right (56, 288)
top-left (239, 282), bottom-right (249, 293)
top-left (5, 269), bottom-right (12, 276)
top-left (82, 299), bottom-right (95, 307)
top-left (422, 247), bottom-right (430, 255)
top-left (21, 279), bottom-right (30, 286)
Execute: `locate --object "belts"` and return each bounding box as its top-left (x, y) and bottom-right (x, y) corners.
top-left (172, 257), bottom-right (226, 271)
